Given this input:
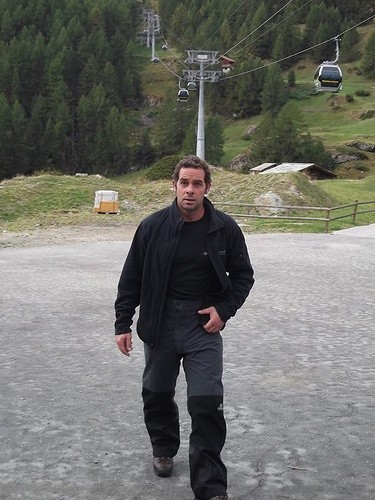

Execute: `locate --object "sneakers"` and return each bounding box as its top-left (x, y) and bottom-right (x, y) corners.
top-left (210, 496), bottom-right (229, 500)
top-left (153, 456), bottom-right (173, 476)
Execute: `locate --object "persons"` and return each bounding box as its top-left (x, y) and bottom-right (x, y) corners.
top-left (114, 153), bottom-right (254, 500)
